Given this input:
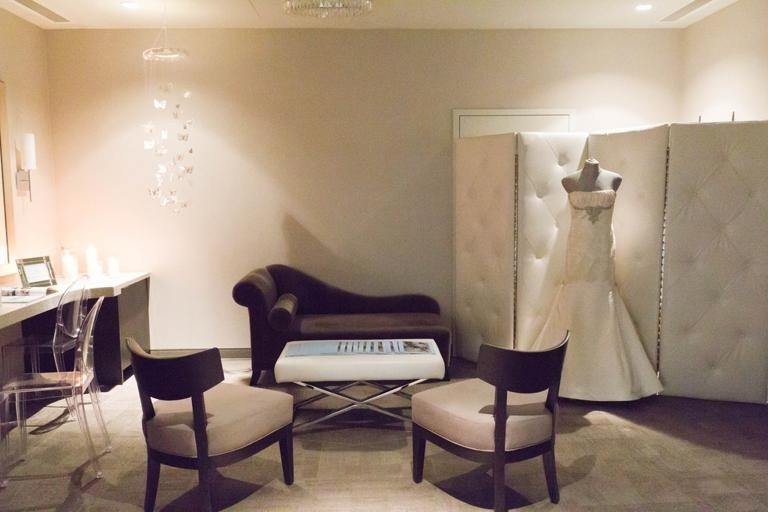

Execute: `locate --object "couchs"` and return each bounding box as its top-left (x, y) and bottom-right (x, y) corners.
top-left (231, 263), bottom-right (452, 386)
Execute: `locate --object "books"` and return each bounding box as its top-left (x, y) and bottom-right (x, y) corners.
top-left (286, 339), bottom-right (436, 356)
top-left (0, 286), bottom-right (58, 303)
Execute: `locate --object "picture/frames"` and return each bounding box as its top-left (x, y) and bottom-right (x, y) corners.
top-left (0, 112), bottom-right (18, 277)
top-left (16, 256), bottom-right (58, 288)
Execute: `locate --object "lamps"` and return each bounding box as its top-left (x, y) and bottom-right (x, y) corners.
top-left (15, 133), bottom-right (36, 202)
top-left (285, 1), bottom-right (374, 19)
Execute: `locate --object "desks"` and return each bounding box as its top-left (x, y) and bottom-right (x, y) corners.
top-left (0, 271), bottom-right (151, 440)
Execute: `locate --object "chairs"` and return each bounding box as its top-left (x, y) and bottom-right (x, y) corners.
top-left (0, 275), bottom-right (101, 422)
top-left (125, 337), bottom-right (293, 512)
top-left (412, 329), bottom-right (571, 512)
top-left (0, 296), bottom-right (112, 487)
